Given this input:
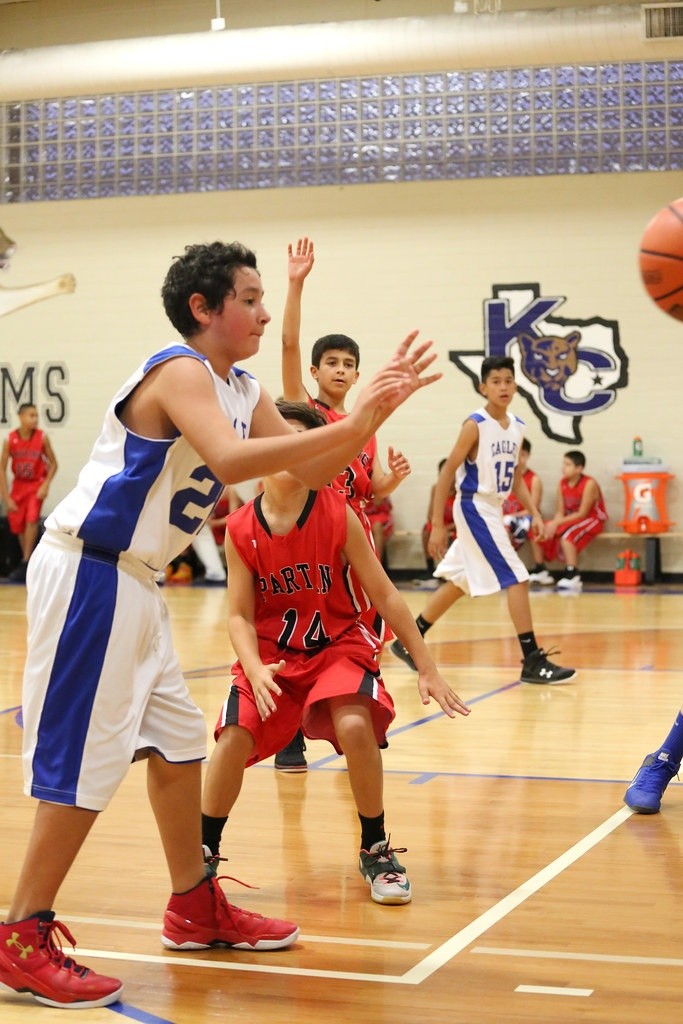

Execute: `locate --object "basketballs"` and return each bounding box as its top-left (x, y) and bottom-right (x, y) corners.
top-left (637, 195), bottom-right (683, 322)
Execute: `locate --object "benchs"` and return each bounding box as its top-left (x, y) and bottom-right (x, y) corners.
top-left (374, 529), bottom-right (682, 585)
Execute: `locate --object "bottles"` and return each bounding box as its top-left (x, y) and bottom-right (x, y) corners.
top-left (633, 437), bottom-right (642, 458)
top-left (617, 552), bottom-right (625, 570)
top-left (630, 553), bottom-right (640, 570)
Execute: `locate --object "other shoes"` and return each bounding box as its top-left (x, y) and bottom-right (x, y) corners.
top-left (412, 570), bottom-right (440, 585)
top-left (9, 561), bottom-right (28, 580)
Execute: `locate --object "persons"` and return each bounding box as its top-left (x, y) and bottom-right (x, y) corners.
top-left (391, 356), bottom-right (576, 685)
top-left (0, 242), bottom-right (442, 1009)
top-left (159, 485), bottom-right (240, 587)
top-left (502, 438), bottom-right (542, 550)
top-left (623, 706), bottom-right (683, 813)
top-left (528, 451), bottom-right (608, 588)
top-left (275, 236), bottom-right (411, 772)
top-left (202, 401), bottom-right (470, 904)
top-left (414, 458), bottom-right (458, 584)
top-left (0, 403), bottom-right (57, 580)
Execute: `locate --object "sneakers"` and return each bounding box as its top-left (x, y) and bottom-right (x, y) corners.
top-left (527, 563), bottom-right (554, 585)
top-left (0, 910), bottom-right (124, 1009)
top-left (556, 566), bottom-right (582, 588)
top-left (274, 730), bottom-right (309, 772)
top-left (201, 845), bottom-right (229, 876)
top-left (171, 561), bottom-right (192, 581)
top-left (623, 748), bottom-right (681, 813)
top-left (520, 645), bottom-right (578, 685)
top-left (162, 563), bottom-right (174, 584)
top-left (161, 876), bottom-right (300, 949)
top-left (391, 638), bottom-right (418, 672)
top-left (360, 832), bottom-right (412, 904)
